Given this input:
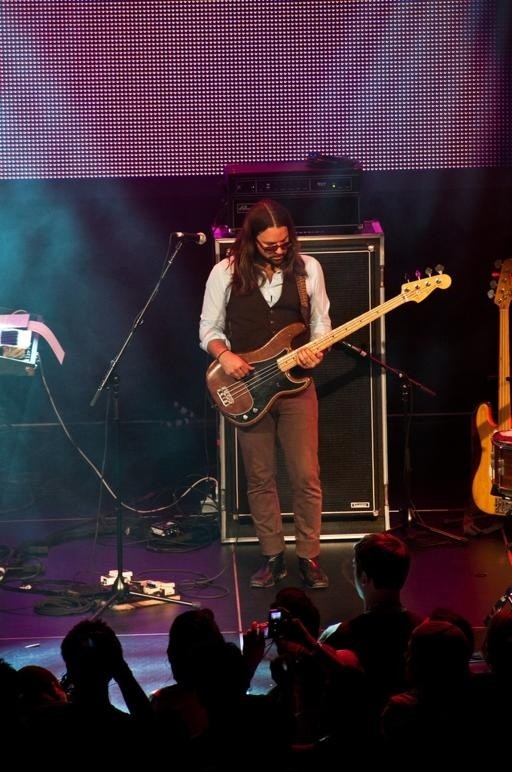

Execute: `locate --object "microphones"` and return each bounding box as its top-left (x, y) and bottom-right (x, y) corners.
top-left (176, 232), bottom-right (207, 245)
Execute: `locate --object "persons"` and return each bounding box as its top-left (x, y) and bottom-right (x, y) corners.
top-left (314, 532), bottom-right (422, 698)
top-left (198, 198), bottom-right (329, 591)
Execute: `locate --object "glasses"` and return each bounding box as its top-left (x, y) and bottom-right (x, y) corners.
top-left (254, 238), bottom-right (292, 254)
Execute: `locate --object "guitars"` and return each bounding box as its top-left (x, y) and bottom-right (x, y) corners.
top-left (205, 265), bottom-right (453, 426)
top-left (472, 259), bottom-right (512, 515)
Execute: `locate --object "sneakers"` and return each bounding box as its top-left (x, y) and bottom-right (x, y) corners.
top-left (249, 552), bottom-right (288, 588)
top-left (297, 553), bottom-right (331, 590)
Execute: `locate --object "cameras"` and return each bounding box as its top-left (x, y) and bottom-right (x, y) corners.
top-left (257, 622), bottom-right (268, 639)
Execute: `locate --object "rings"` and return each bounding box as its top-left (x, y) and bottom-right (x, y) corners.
top-left (303, 362), bottom-right (308, 366)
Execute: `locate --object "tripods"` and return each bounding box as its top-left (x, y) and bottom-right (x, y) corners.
top-left (338, 341), bottom-right (471, 547)
top-left (66, 246), bottom-right (202, 624)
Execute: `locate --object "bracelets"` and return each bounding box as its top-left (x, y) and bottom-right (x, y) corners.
top-left (217, 348), bottom-right (228, 360)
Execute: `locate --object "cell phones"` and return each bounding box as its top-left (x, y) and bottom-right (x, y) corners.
top-left (268, 609), bottom-right (283, 638)
